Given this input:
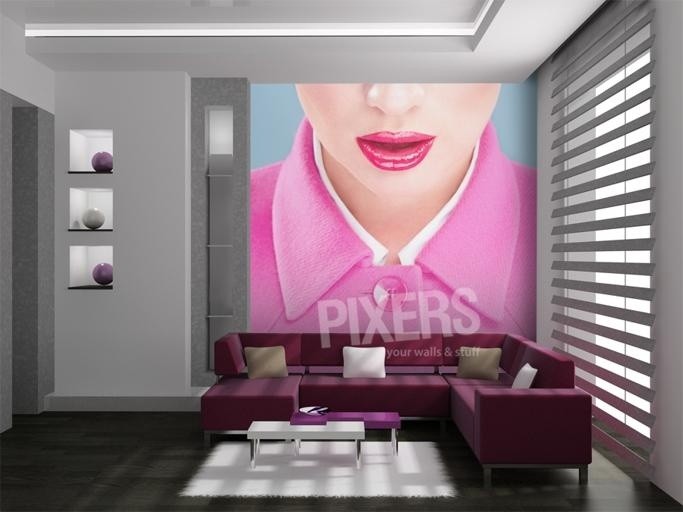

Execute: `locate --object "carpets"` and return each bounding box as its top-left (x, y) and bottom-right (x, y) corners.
top-left (178, 442), bottom-right (456, 499)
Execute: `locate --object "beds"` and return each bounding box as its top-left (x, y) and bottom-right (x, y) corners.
top-left (200, 333), bottom-right (303, 449)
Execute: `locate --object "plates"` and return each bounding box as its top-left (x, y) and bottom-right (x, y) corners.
top-left (299, 405), bottom-right (330, 416)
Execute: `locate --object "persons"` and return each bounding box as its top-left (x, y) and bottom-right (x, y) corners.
top-left (246, 81), bottom-right (538, 342)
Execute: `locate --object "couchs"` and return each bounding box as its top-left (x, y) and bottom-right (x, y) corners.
top-left (300, 334), bottom-right (450, 437)
top-left (443, 332), bottom-right (591, 491)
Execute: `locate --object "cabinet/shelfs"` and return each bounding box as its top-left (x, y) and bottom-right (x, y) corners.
top-left (68, 129), bottom-right (114, 291)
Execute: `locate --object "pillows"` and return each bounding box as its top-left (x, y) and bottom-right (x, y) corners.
top-left (243, 345), bottom-right (287, 379)
top-left (458, 346), bottom-right (502, 381)
top-left (342, 346), bottom-right (387, 379)
top-left (511, 363), bottom-right (538, 390)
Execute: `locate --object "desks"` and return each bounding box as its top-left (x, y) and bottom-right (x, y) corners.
top-left (292, 411), bottom-right (403, 456)
top-left (247, 421), bottom-right (366, 471)
